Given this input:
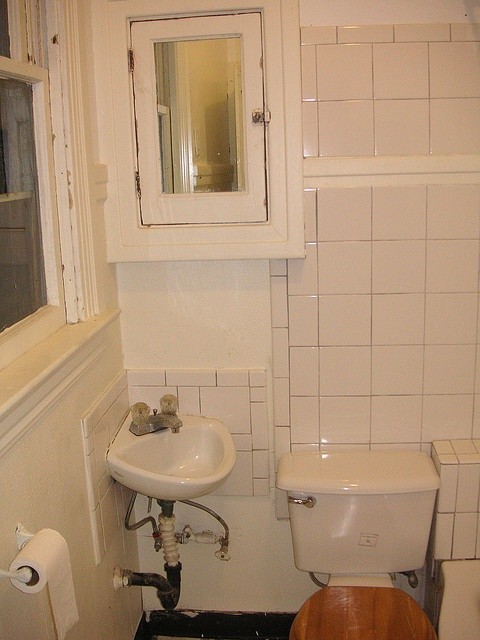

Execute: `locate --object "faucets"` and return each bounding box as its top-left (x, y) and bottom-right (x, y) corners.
top-left (129, 394), bottom-right (182, 435)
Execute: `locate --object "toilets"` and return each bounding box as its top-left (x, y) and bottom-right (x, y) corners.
top-left (276, 448), bottom-right (441, 640)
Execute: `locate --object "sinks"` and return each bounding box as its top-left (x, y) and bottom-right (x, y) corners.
top-left (108, 411), bottom-right (237, 500)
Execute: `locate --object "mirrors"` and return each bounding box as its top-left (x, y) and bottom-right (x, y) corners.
top-left (130, 18), bottom-right (266, 224)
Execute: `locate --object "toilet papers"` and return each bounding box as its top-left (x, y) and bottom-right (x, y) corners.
top-left (10, 527), bottom-right (80, 640)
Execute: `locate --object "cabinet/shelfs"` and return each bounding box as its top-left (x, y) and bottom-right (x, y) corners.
top-left (91, 1), bottom-right (302, 265)
